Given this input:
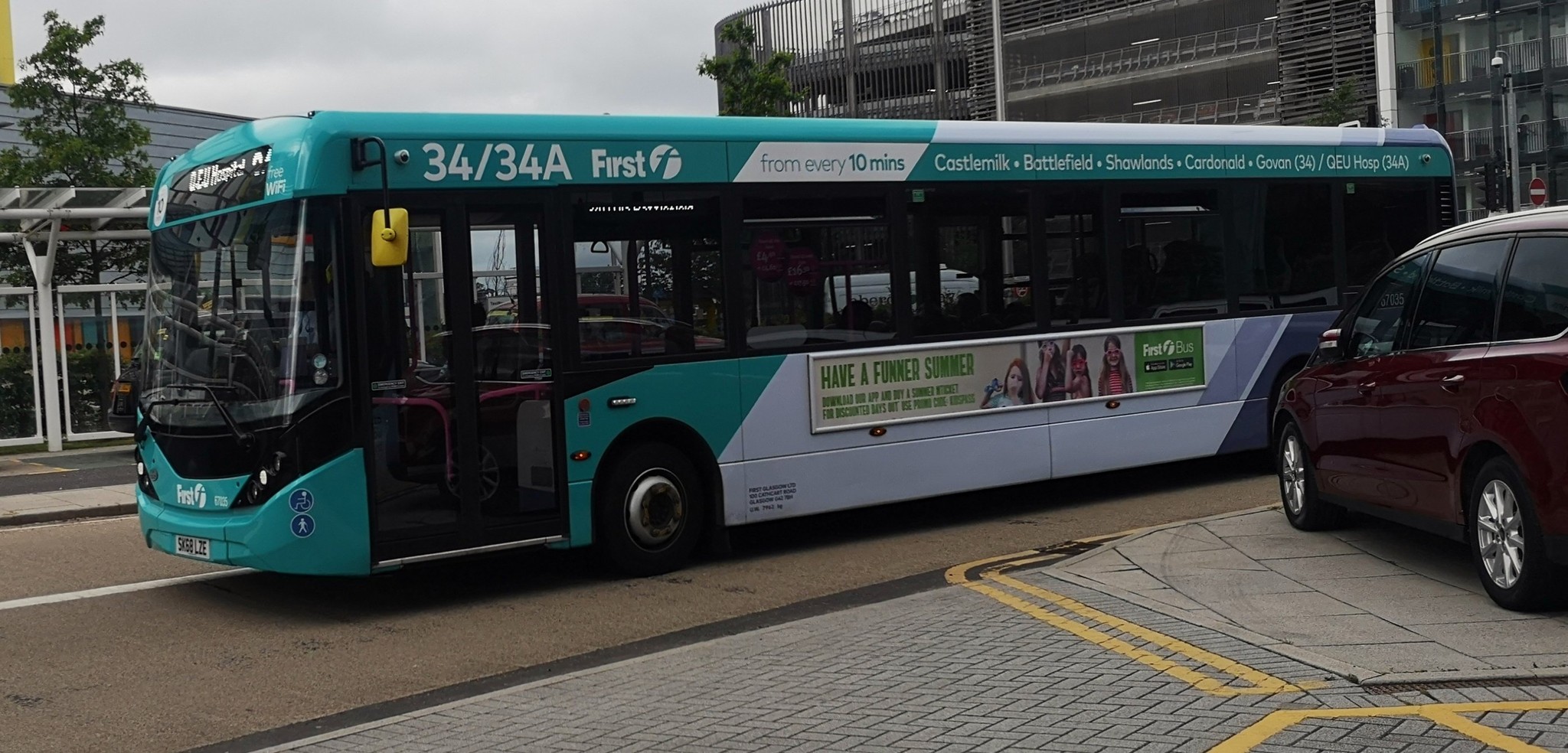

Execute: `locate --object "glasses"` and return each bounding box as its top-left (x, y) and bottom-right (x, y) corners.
top-left (1041, 341), bottom-right (1054, 350)
top-left (1073, 359), bottom-right (1086, 368)
top-left (1105, 348), bottom-right (1120, 355)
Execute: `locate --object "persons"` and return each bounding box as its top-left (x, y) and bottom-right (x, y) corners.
top-left (442, 302), bottom-right (499, 387)
top-left (1098, 335), bottom-right (1133, 396)
top-left (660, 215), bottom-right (1395, 341)
top-left (981, 359), bottom-right (1034, 410)
top-left (299, 260), bottom-right (351, 347)
top-left (1065, 344), bottom-right (1092, 399)
top-left (1518, 115), bottom-right (1534, 150)
top-left (1035, 338), bottom-right (1071, 403)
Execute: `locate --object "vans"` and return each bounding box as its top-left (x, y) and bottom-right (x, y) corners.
top-left (817, 265), bottom-right (986, 322)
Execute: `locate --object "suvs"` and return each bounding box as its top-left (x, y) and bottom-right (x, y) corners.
top-left (1269, 203), bottom-right (1567, 618)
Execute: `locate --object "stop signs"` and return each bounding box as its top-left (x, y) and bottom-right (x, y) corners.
top-left (1530, 178), bottom-right (1546, 205)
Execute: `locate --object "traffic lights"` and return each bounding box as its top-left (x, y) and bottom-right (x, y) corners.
top-left (1474, 160), bottom-right (1500, 212)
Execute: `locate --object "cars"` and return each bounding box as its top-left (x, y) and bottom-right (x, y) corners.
top-left (515, 294), bottom-right (732, 353)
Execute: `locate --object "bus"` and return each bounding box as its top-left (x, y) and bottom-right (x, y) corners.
top-left (125, 112), bottom-right (1462, 575)
